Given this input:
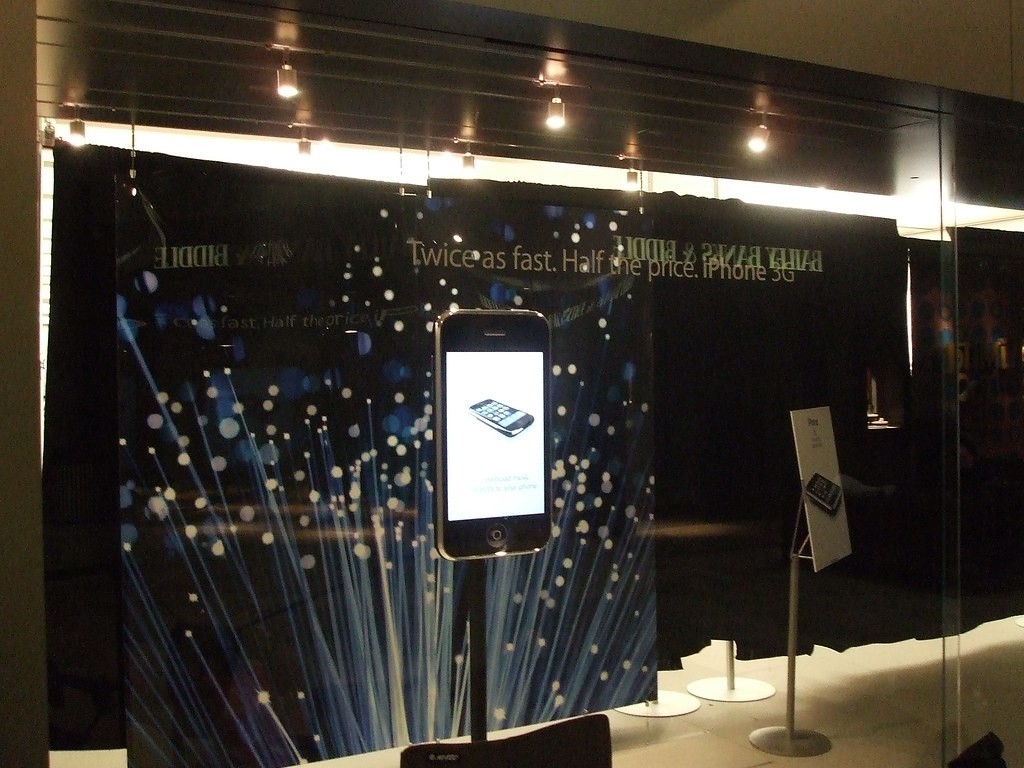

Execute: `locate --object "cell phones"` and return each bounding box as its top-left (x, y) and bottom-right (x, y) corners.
top-left (433, 306), bottom-right (552, 563)
top-left (806, 472), bottom-right (844, 515)
top-left (467, 397), bottom-right (535, 437)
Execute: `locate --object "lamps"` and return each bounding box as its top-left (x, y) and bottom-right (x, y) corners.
top-left (626, 169), bottom-right (640, 190)
top-left (546, 86), bottom-right (566, 128)
top-left (462, 154), bottom-right (475, 174)
top-left (298, 139), bottom-right (311, 160)
top-left (276, 48), bottom-right (298, 98)
top-left (747, 116), bottom-right (771, 152)
